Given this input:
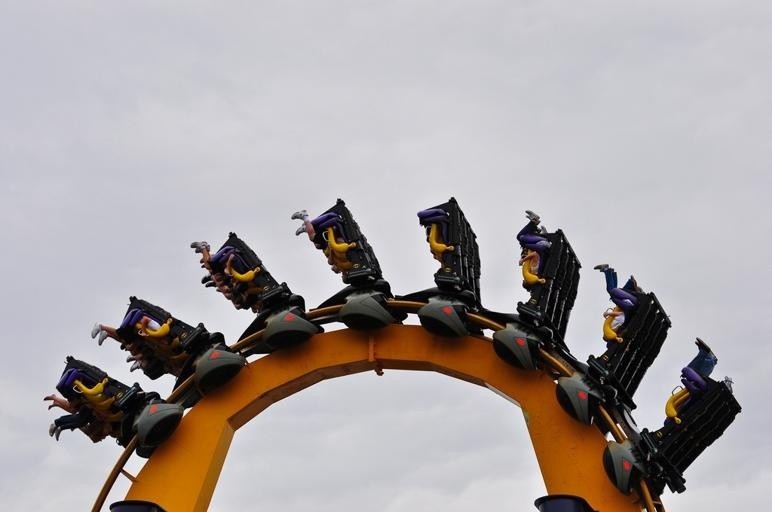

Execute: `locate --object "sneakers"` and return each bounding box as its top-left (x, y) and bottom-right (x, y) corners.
top-left (201, 275), bottom-right (217, 287)
top-left (49, 424), bottom-right (61, 441)
top-left (593, 264), bottom-right (615, 272)
top-left (291, 210), bottom-right (308, 219)
top-left (92, 322), bottom-right (108, 346)
top-left (525, 210), bottom-right (540, 225)
top-left (695, 337), bottom-right (718, 361)
top-left (190, 241), bottom-right (210, 253)
top-left (541, 225), bottom-right (546, 235)
top-left (296, 222), bottom-right (307, 236)
top-left (127, 355), bottom-right (142, 372)
top-left (200, 258), bottom-right (207, 268)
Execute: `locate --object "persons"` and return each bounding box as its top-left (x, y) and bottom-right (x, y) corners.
top-left (594, 263), bottom-right (640, 349)
top-left (290, 209), bottom-right (355, 274)
top-left (91, 310), bottom-right (178, 380)
top-left (686, 336), bottom-right (718, 378)
top-left (517, 209), bottom-right (548, 293)
top-left (43, 395), bottom-right (115, 441)
top-left (190, 241), bottom-right (263, 314)
